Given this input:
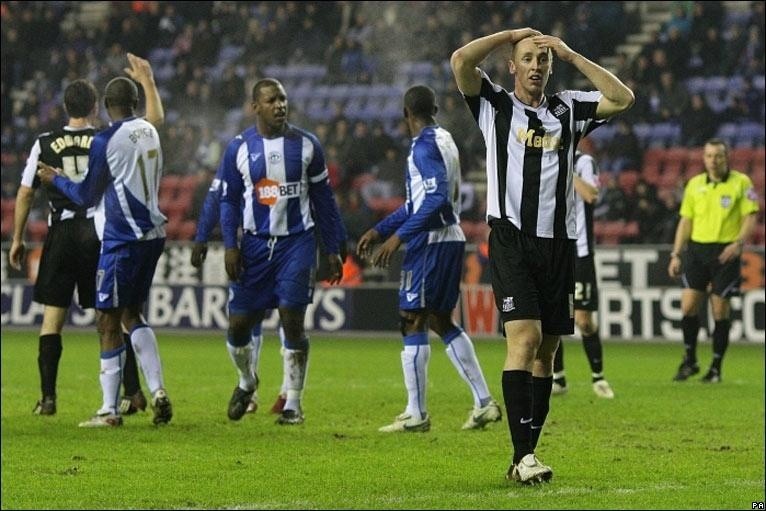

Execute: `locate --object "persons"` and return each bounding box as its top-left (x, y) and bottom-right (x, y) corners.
top-left (37, 77), bottom-right (172, 426)
top-left (162, 127), bottom-right (188, 174)
top-left (186, 173), bottom-right (212, 218)
top-left (452, 0), bottom-right (761, 31)
top-left (221, 77), bottom-right (348, 422)
top-left (192, 125), bottom-right (219, 175)
top-left (630, 1), bottom-right (762, 107)
top-left (551, 148), bottom-right (615, 400)
top-left (189, 143), bottom-right (329, 415)
top-left (450, 28), bottom-right (635, 485)
top-left (20, 119), bottom-right (40, 162)
top-left (8, 53), bottom-right (164, 416)
top-left (637, 180), bottom-right (680, 242)
top-left (1, 129), bottom-right (21, 197)
top-left (0, 0), bottom-right (451, 121)
top-left (636, 32), bottom-right (761, 145)
top-left (349, 84), bottom-right (503, 433)
top-left (668, 138), bottom-right (759, 383)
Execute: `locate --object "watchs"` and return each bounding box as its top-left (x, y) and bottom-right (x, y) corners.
top-left (734, 240), bottom-right (746, 245)
top-left (670, 253), bottom-right (681, 258)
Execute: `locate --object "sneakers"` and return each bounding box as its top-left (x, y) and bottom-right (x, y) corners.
top-left (506, 464), bottom-right (518, 480)
top-left (672, 363), bottom-right (699, 381)
top-left (271, 393), bottom-right (286, 412)
top-left (77, 409), bottom-right (122, 427)
top-left (33, 397), bottom-right (56, 416)
top-left (228, 376), bottom-right (258, 421)
top-left (592, 380), bottom-right (613, 399)
top-left (462, 401), bottom-right (501, 431)
top-left (379, 411), bottom-right (432, 432)
top-left (700, 371), bottom-right (720, 384)
top-left (119, 391), bottom-right (146, 414)
top-left (548, 377), bottom-right (568, 394)
top-left (151, 389), bottom-right (173, 424)
top-left (516, 455), bottom-right (551, 484)
top-left (275, 408), bottom-right (304, 425)
top-left (245, 392), bottom-right (257, 412)
